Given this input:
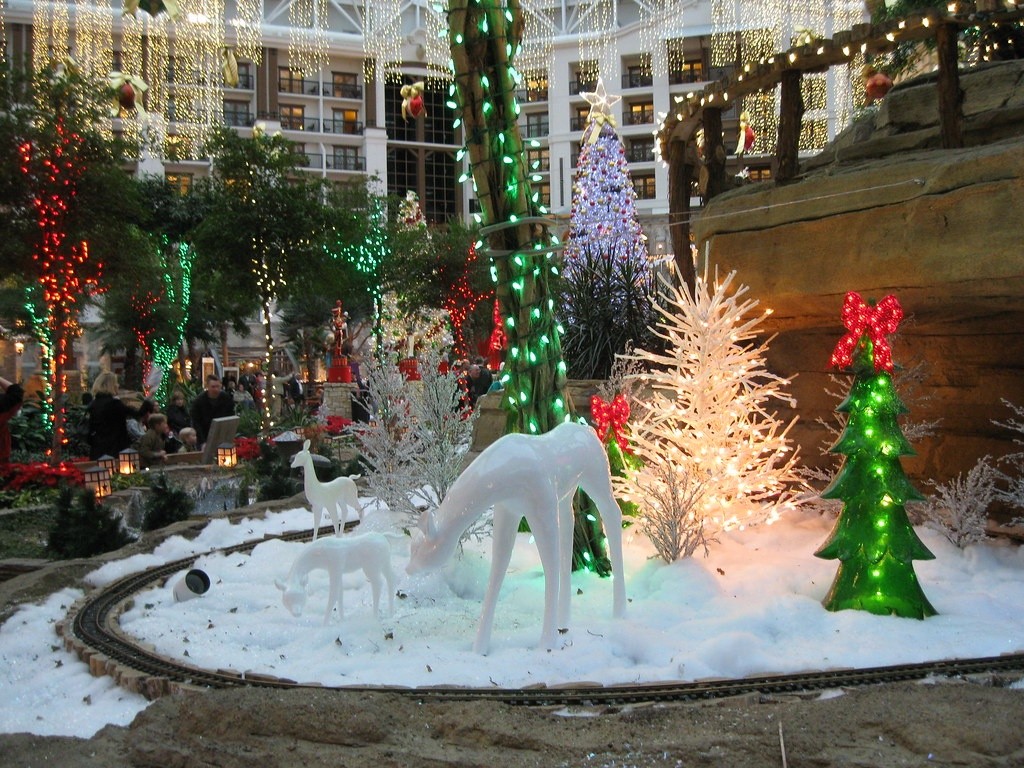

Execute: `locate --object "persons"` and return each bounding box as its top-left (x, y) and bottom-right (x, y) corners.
top-left (0, 376), bottom-right (25, 463)
top-left (463, 356), bottom-right (492, 411)
top-left (89, 363), bottom-right (363, 470)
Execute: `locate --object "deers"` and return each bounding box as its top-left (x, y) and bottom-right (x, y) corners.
top-left (275, 531), bottom-right (405, 626)
top-left (405, 422), bottom-right (626, 657)
top-left (290, 438), bottom-right (365, 543)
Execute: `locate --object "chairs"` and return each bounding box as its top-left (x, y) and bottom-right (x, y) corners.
top-left (243, 78), bottom-right (364, 170)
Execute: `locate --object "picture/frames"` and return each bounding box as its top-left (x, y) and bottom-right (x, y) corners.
top-left (201, 356), bottom-right (215, 390)
top-left (222, 366), bottom-right (239, 390)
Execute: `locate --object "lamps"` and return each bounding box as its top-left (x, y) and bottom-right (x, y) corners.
top-left (272, 430), bottom-right (303, 457)
top-left (216, 442), bottom-right (237, 467)
top-left (84, 464), bottom-right (111, 499)
top-left (97, 455), bottom-right (117, 477)
top-left (119, 448), bottom-right (139, 474)
top-left (173, 568), bottom-right (211, 601)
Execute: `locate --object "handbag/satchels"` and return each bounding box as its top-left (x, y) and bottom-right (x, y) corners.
top-left (77, 400), bottom-right (95, 445)
